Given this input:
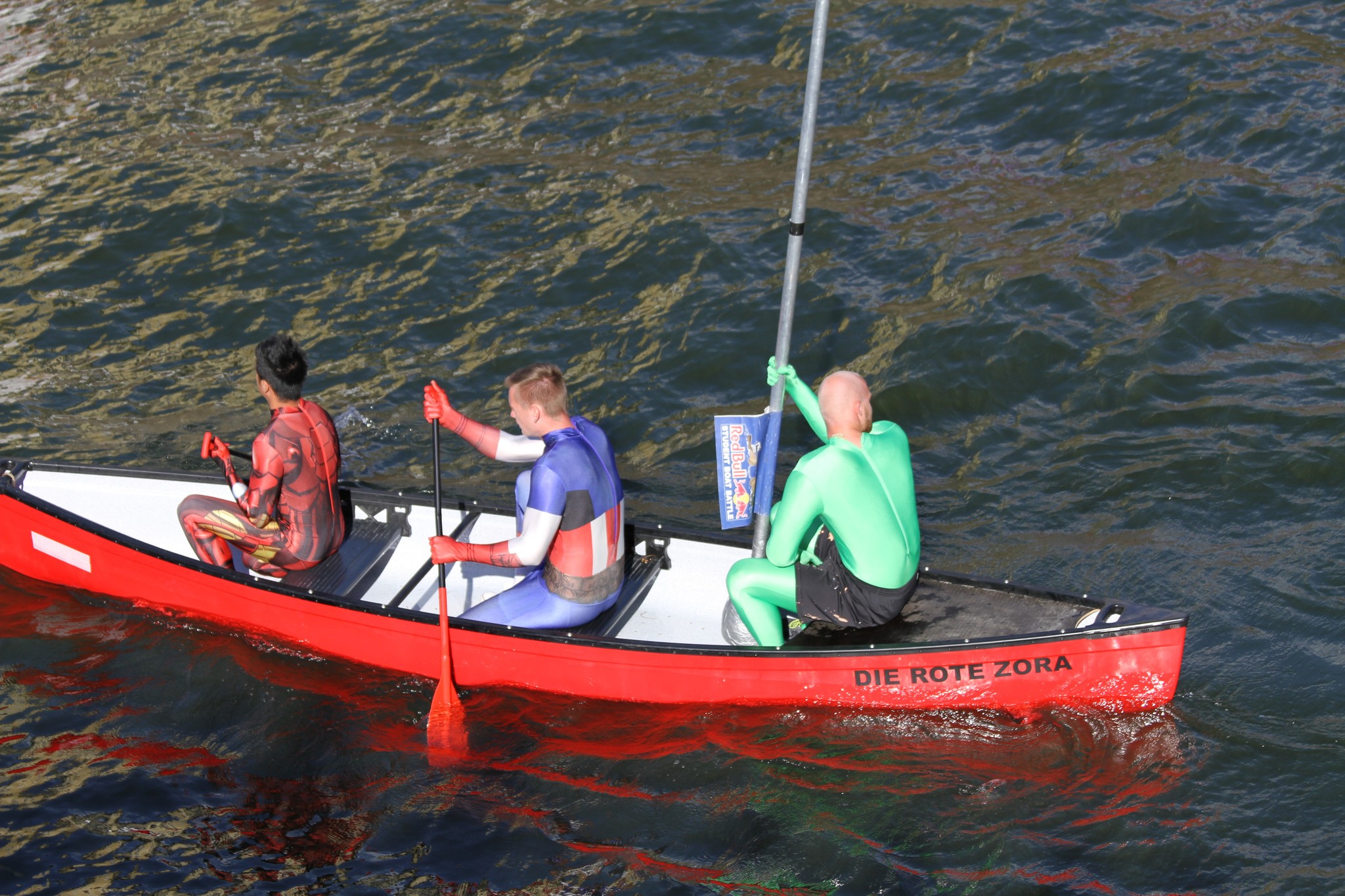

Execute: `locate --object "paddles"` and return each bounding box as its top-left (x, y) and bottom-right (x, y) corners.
top-left (427, 383), bottom-right (466, 773)
top-left (202, 428), bottom-right (253, 463)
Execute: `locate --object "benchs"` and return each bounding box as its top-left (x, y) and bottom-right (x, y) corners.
top-left (278, 498), bottom-right (673, 640)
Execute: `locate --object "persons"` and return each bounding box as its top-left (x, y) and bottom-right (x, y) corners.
top-left (423, 364), bottom-right (626, 628)
top-left (725, 356), bottom-right (921, 647)
top-left (177, 335), bottom-right (345, 578)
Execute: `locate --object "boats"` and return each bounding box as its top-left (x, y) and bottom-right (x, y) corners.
top-left (0, 458), bottom-right (1190, 722)
